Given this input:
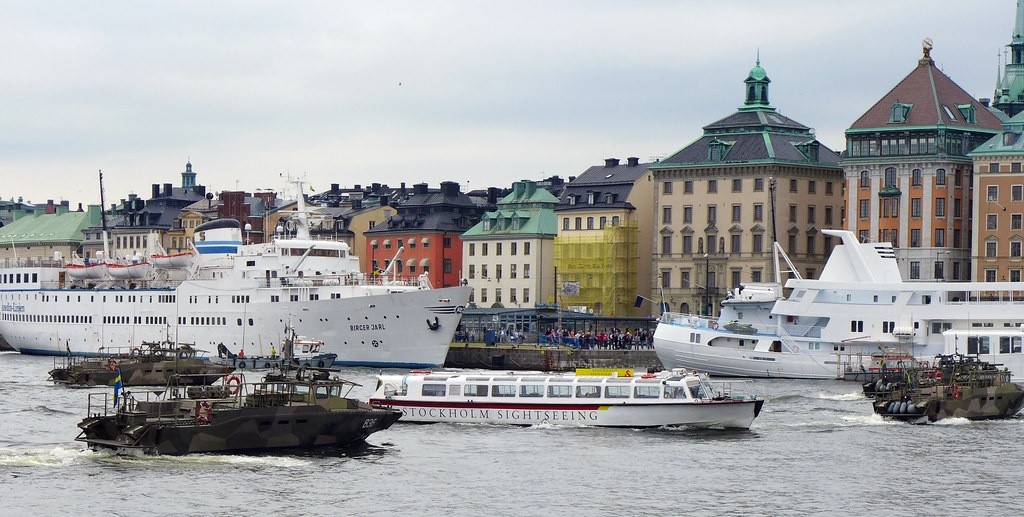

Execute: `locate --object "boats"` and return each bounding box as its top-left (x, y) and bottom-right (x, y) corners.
top-left (107, 262), bottom-right (149, 280)
top-left (74, 329), bottom-right (402, 459)
top-left (653, 229), bottom-right (1024, 382)
top-left (0, 169), bottom-right (472, 368)
top-left (151, 252), bottom-right (193, 269)
top-left (46, 340), bottom-right (236, 389)
top-left (863, 354), bottom-right (1024, 424)
top-left (65, 263), bottom-right (106, 279)
top-left (369, 367), bottom-right (765, 430)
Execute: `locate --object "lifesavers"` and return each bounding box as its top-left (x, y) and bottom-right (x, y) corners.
top-left (265, 362), bottom-right (271, 368)
top-left (642, 373), bottom-right (656, 378)
top-left (109, 360), bottom-right (116, 369)
top-left (935, 372), bottom-right (943, 382)
top-left (132, 348), bottom-right (140, 357)
top-left (179, 351), bottom-right (188, 359)
top-left (226, 376), bottom-right (241, 395)
top-left (712, 321), bottom-right (718, 330)
top-left (196, 403), bottom-right (213, 424)
top-left (239, 362), bottom-right (246, 368)
top-left (954, 384), bottom-right (959, 398)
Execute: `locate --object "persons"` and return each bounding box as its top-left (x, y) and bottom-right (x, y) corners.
top-left (739, 285), bottom-right (744, 294)
top-left (543, 327), bottom-right (653, 350)
top-left (453, 326), bottom-right (525, 344)
top-left (272, 346), bottom-right (276, 354)
top-left (238, 349), bottom-right (247, 359)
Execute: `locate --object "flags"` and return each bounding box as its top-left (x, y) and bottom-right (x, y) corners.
top-left (113, 370), bottom-right (122, 407)
top-left (634, 296), bottom-right (643, 308)
